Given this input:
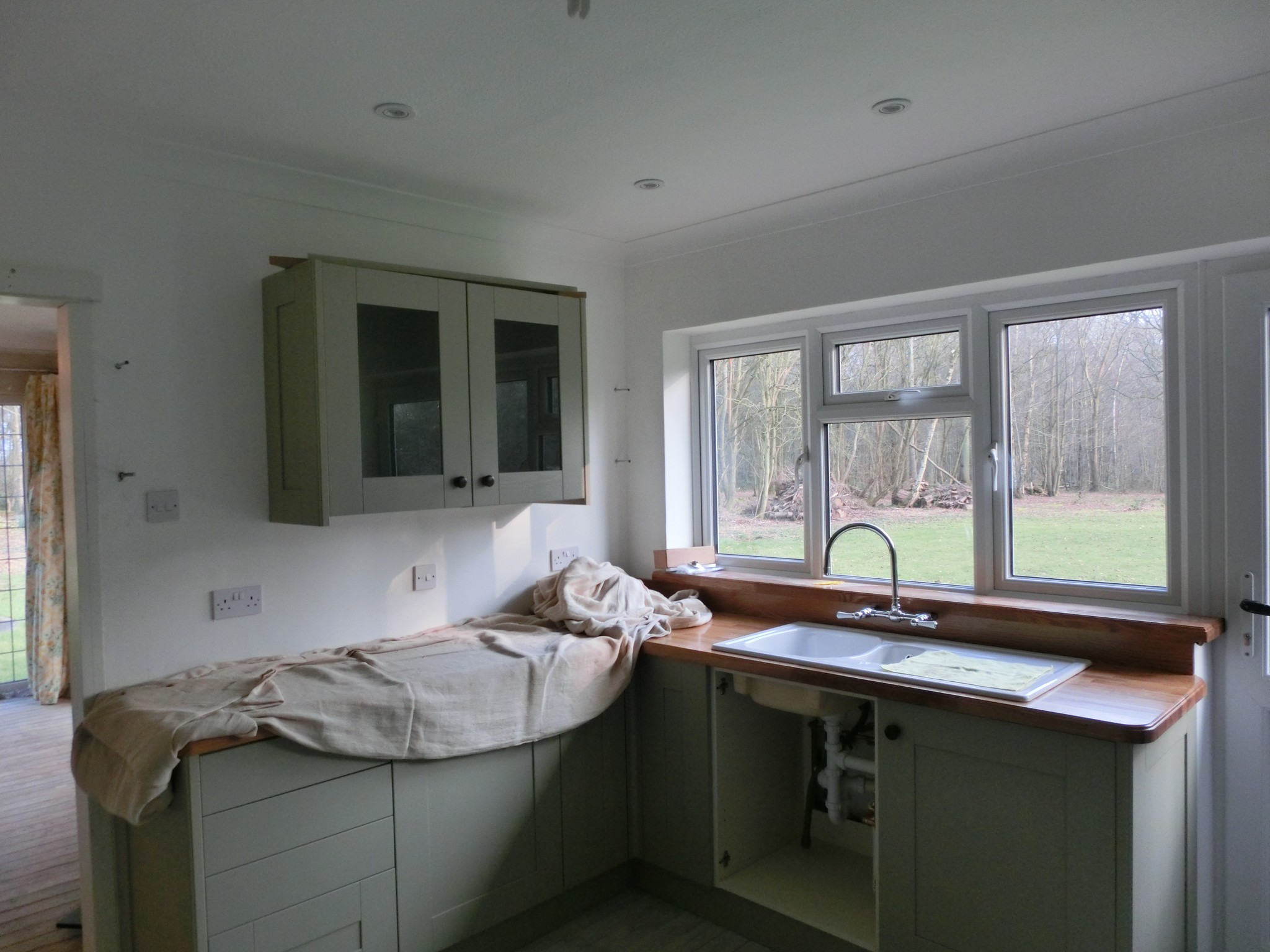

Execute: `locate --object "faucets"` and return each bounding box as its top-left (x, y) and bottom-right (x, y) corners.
top-left (821, 522), bottom-right (939, 630)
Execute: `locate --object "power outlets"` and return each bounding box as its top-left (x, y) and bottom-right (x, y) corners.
top-left (212, 585), bottom-right (262, 619)
top-left (551, 546), bottom-right (580, 571)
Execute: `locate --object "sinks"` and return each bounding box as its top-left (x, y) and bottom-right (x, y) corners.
top-left (846, 643), bottom-right (929, 674)
top-left (711, 619), bottom-right (891, 718)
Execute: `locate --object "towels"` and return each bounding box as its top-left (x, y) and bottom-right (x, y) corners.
top-left (880, 649), bottom-right (1053, 692)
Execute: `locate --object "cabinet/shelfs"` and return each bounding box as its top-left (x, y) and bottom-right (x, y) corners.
top-left (879, 699), bottom-right (1185, 952)
top-left (261, 259), bottom-right (591, 527)
top-left (112, 652), bottom-right (713, 952)
